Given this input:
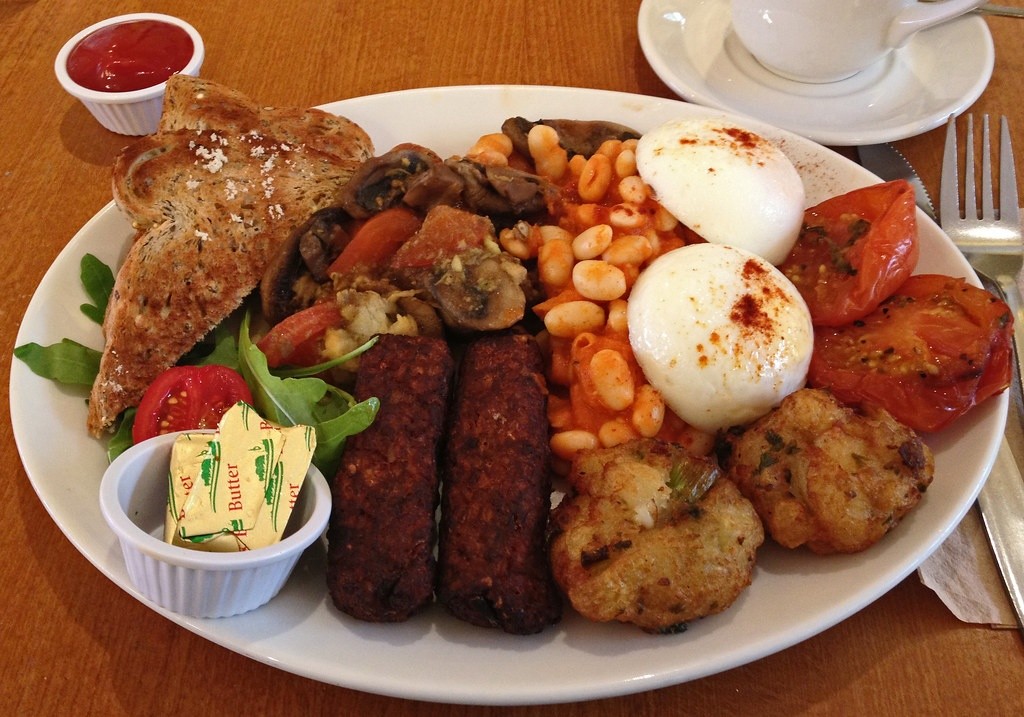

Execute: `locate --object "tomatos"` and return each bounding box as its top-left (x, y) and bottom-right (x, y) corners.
top-left (777, 179), bottom-right (1014, 436)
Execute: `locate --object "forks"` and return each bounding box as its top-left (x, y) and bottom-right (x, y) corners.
top-left (939, 112), bottom-right (1024, 397)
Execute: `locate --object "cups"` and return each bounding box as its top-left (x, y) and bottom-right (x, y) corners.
top-left (730, 0), bottom-right (989, 85)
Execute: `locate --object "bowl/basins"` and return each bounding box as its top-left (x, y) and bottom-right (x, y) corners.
top-left (54, 11), bottom-right (206, 138)
top-left (97, 431), bottom-right (332, 620)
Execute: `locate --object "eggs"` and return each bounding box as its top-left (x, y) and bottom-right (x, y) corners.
top-left (626, 118), bottom-right (815, 432)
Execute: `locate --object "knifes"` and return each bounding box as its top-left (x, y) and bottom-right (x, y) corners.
top-left (857, 145), bottom-right (1024, 630)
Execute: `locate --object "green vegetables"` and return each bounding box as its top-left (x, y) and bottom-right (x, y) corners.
top-left (12, 250), bottom-right (380, 466)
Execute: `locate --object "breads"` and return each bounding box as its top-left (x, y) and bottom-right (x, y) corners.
top-left (88, 73), bottom-right (376, 446)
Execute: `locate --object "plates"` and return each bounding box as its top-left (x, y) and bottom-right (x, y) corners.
top-left (636, 0), bottom-right (995, 146)
top-left (9, 84), bottom-right (1009, 707)
